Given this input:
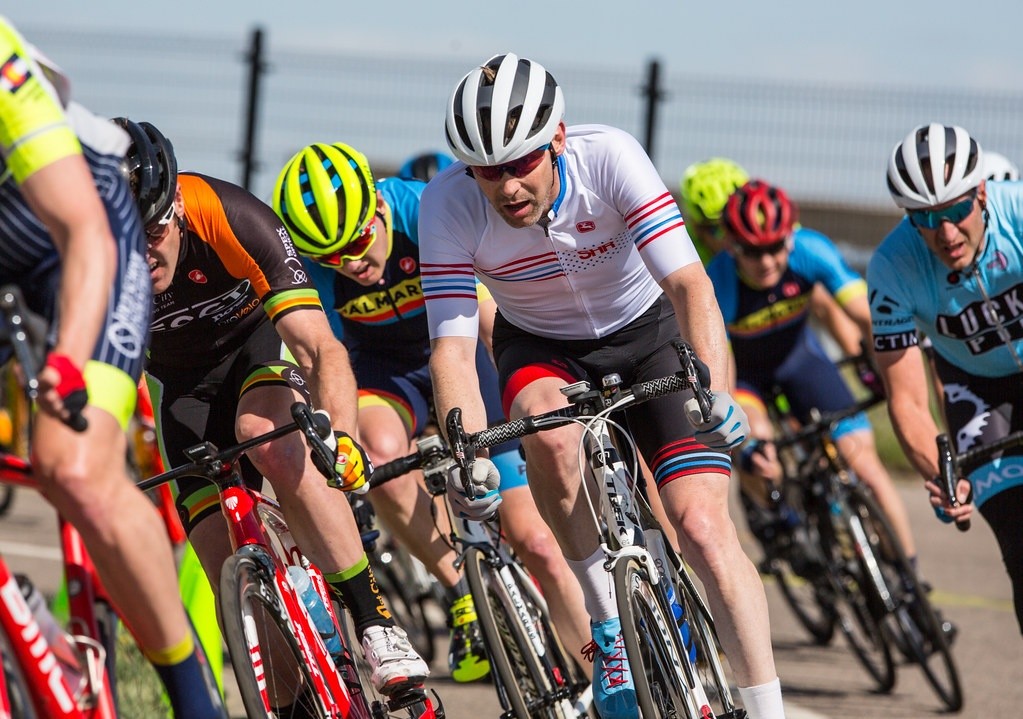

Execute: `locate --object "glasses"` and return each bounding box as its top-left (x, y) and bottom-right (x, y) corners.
top-left (469, 151), bottom-right (545, 182)
top-left (735, 238), bottom-right (786, 258)
top-left (905, 186), bottom-right (977, 230)
top-left (312, 224), bottom-right (377, 268)
top-left (143, 200), bottom-right (175, 250)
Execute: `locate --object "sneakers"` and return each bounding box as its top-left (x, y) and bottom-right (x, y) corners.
top-left (590, 616), bottom-right (640, 719)
top-left (447, 593), bottom-right (491, 682)
top-left (358, 625), bottom-right (431, 696)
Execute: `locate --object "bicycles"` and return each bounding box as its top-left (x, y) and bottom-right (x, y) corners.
top-left (128, 402), bottom-right (445, 719)
top-left (351, 339), bottom-right (747, 719)
top-left (0, 282), bottom-right (230, 719)
top-left (929, 432), bottom-right (1023, 531)
top-left (729, 341), bottom-right (966, 713)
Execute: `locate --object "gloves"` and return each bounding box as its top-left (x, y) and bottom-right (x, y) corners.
top-left (445, 457), bottom-right (503, 521)
top-left (310, 429), bottom-right (375, 492)
top-left (684, 390), bottom-right (751, 454)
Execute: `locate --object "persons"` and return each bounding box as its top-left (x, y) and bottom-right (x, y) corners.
top-left (110, 114), bottom-right (433, 701)
top-left (0, 11), bottom-right (234, 719)
top-left (867, 124), bottom-right (1023, 635)
top-left (983, 148), bottom-right (1023, 183)
top-left (414, 54), bottom-right (790, 719)
top-left (270, 139), bottom-right (506, 686)
top-left (679, 158), bottom-right (960, 665)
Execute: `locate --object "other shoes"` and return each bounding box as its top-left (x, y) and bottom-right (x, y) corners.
top-left (898, 580), bottom-right (952, 639)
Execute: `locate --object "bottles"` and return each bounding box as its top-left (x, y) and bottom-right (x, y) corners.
top-left (284, 563), bottom-right (344, 659)
top-left (654, 557), bottom-right (697, 664)
top-left (12, 573), bottom-right (91, 689)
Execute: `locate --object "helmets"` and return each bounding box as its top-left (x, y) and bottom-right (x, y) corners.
top-left (444, 52), bottom-right (564, 166)
top-left (397, 152), bottom-right (454, 185)
top-left (679, 156), bottom-right (750, 226)
top-left (983, 151), bottom-right (1019, 182)
top-left (720, 179), bottom-right (800, 247)
top-left (271, 141), bottom-right (378, 260)
top-left (109, 116), bottom-right (178, 229)
top-left (886, 122), bottom-right (989, 209)
